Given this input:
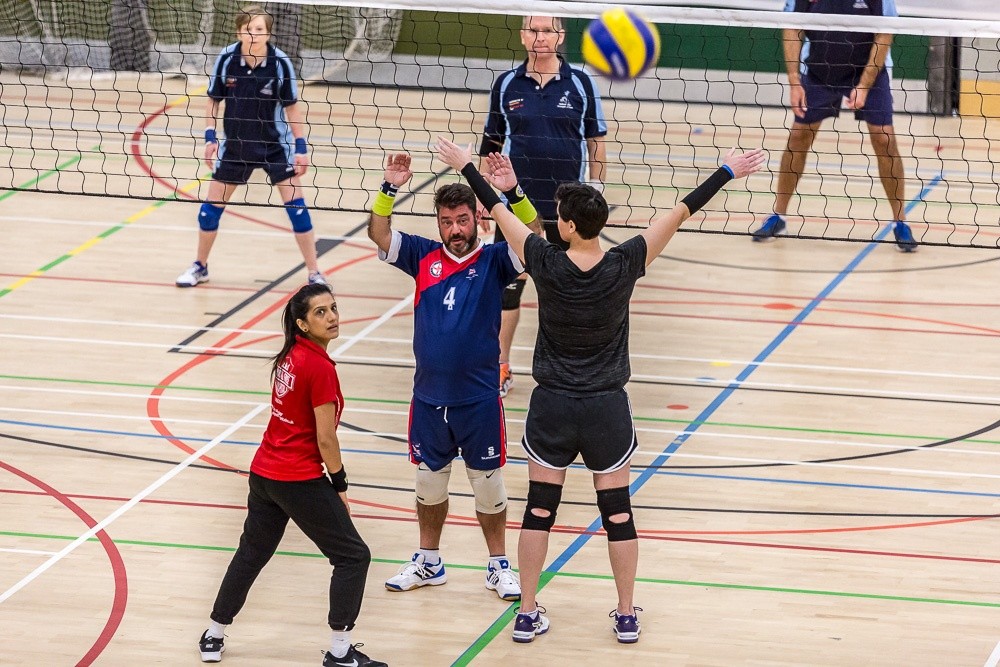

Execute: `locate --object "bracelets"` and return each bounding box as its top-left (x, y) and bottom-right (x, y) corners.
top-left (327, 463), bottom-right (348, 493)
top-left (502, 182), bottom-right (538, 225)
top-left (203, 126), bottom-right (217, 144)
top-left (372, 179), bottom-right (403, 217)
top-left (293, 137), bottom-right (308, 154)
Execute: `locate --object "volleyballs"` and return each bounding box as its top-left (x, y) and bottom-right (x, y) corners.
top-left (578, 8), bottom-right (661, 83)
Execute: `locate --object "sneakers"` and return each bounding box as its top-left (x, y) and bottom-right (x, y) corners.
top-left (385, 552), bottom-right (447, 592)
top-left (176, 261), bottom-right (209, 287)
top-left (499, 363), bottom-right (515, 398)
top-left (197, 629), bottom-right (229, 661)
top-left (320, 642), bottom-right (388, 667)
top-left (753, 213), bottom-right (788, 242)
top-left (892, 220), bottom-right (919, 252)
top-left (308, 271), bottom-right (329, 289)
top-left (486, 560), bottom-right (522, 601)
top-left (609, 607), bottom-right (643, 643)
top-left (513, 602), bottom-right (550, 643)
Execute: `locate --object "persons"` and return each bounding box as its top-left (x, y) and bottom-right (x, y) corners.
top-left (433, 135), bottom-right (765, 643)
top-left (751, 0), bottom-right (917, 253)
top-left (476, 15), bottom-right (609, 398)
top-left (177, 4), bottom-right (330, 291)
top-left (198, 284), bottom-right (389, 667)
top-left (368, 151), bottom-right (541, 600)
